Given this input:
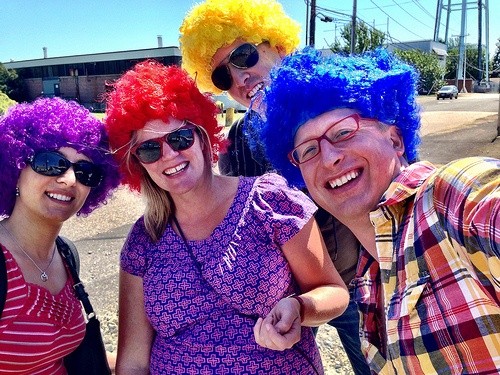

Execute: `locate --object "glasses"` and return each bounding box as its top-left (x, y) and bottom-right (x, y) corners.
top-left (130, 124), bottom-right (194, 164)
top-left (288, 114), bottom-right (377, 166)
top-left (211, 39), bottom-right (266, 91)
top-left (25, 149), bottom-right (104, 188)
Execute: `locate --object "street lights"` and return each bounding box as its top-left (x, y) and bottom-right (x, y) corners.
top-left (309, 12), bottom-right (333, 48)
top-left (455, 33), bottom-right (470, 88)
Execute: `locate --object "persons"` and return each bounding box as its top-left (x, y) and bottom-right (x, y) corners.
top-left (97, 66), bottom-right (349, 375)
top-left (243, 47), bottom-right (500, 375)
top-left (178, 0), bottom-right (375, 375)
top-left (0, 97), bottom-right (121, 375)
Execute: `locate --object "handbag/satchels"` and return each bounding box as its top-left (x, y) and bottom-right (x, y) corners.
top-left (57, 237), bottom-right (112, 375)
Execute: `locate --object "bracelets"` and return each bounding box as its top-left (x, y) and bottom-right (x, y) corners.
top-left (287, 293), bottom-right (304, 323)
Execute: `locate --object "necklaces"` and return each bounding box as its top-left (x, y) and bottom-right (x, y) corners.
top-left (0, 223), bottom-right (57, 282)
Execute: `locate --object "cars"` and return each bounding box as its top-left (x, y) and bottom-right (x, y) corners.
top-left (437, 85), bottom-right (459, 100)
